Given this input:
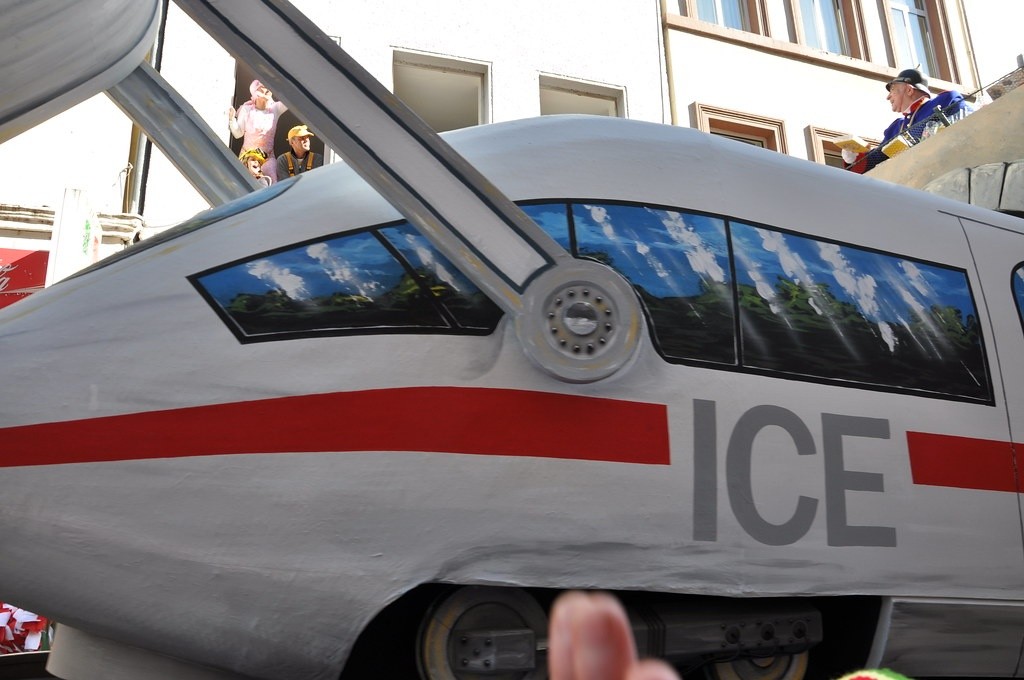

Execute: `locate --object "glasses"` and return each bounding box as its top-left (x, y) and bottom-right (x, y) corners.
top-left (248, 148), bottom-right (268, 159)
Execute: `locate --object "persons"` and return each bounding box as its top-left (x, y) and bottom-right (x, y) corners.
top-left (239, 147), bottom-right (272, 188)
top-left (230, 78), bottom-right (289, 183)
top-left (276, 125), bottom-right (323, 183)
top-left (841, 65), bottom-right (969, 175)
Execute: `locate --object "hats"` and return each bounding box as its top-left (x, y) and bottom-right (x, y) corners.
top-left (250, 80), bottom-right (263, 95)
top-left (286, 126), bottom-right (314, 141)
top-left (240, 150), bottom-right (265, 166)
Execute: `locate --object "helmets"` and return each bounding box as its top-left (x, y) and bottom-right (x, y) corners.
top-left (886, 64), bottom-right (931, 98)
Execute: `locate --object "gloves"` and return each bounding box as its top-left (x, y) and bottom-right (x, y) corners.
top-left (842, 147), bottom-right (859, 164)
